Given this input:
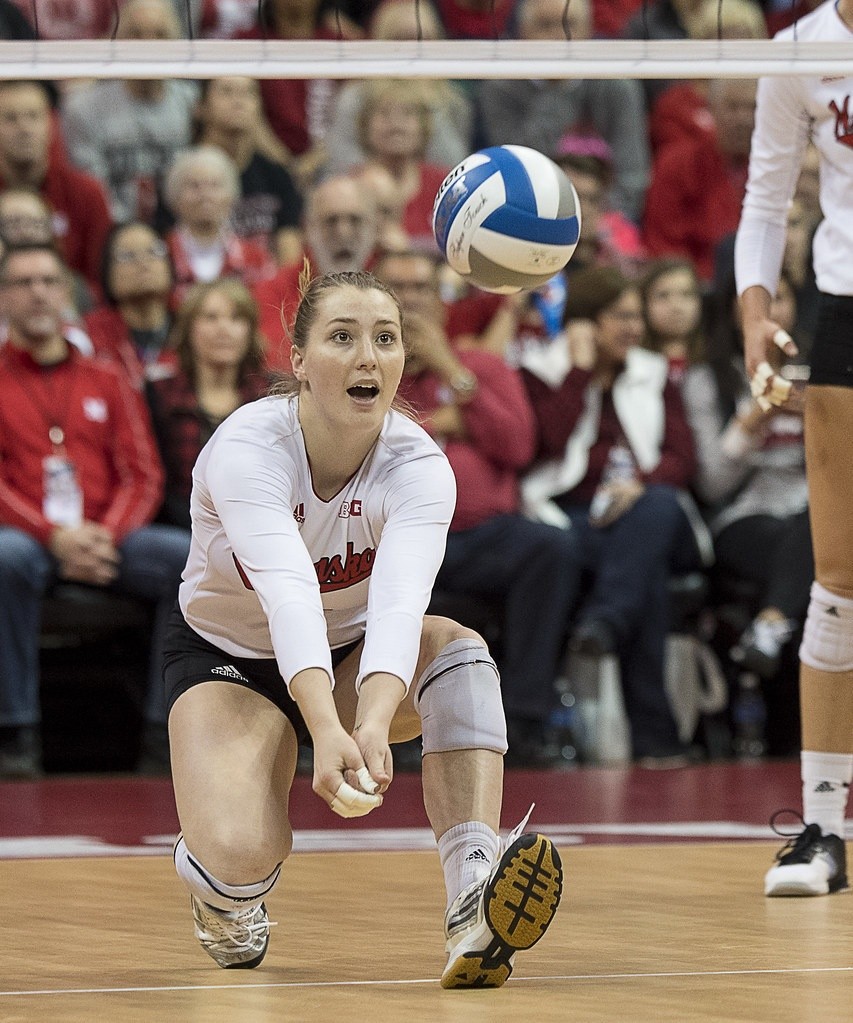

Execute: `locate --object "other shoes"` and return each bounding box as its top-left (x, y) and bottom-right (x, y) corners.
top-left (732, 620), bottom-right (794, 679)
top-left (641, 740), bottom-right (693, 766)
top-left (576, 618), bottom-right (612, 656)
top-left (1, 750), bottom-right (45, 781)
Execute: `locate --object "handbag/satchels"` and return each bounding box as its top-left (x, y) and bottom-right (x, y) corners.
top-left (565, 634), bottom-right (726, 765)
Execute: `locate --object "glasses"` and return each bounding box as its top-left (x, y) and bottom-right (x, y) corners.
top-left (114, 238), bottom-right (169, 263)
top-left (6, 212), bottom-right (68, 240)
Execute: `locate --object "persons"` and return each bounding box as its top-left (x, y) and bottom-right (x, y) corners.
top-left (0, 0), bottom-right (853, 898)
top-left (164, 260), bottom-right (563, 991)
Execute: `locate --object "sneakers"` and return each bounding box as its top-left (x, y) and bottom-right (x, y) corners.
top-left (173, 831), bottom-right (278, 969)
top-left (764, 808), bottom-right (849, 896)
top-left (440, 804), bottom-right (565, 989)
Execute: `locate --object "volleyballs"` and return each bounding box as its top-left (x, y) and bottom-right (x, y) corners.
top-left (432, 144), bottom-right (582, 296)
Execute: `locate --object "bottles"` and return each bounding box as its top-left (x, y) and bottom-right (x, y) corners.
top-left (41, 454), bottom-right (85, 532)
top-left (546, 677), bottom-right (576, 768)
top-left (732, 671), bottom-right (768, 759)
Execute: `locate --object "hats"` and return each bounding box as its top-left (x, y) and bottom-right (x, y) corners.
top-left (560, 133), bottom-right (610, 162)
